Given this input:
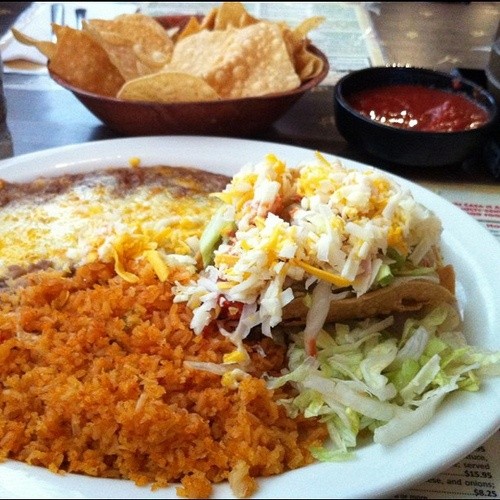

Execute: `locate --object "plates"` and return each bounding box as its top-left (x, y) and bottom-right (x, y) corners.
top-left (0, 137), bottom-right (500, 500)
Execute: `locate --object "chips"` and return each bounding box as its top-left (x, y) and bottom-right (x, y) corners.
top-left (12, 0), bottom-right (323, 102)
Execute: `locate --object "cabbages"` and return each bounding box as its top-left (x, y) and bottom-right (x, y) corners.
top-left (268, 307), bottom-right (500, 462)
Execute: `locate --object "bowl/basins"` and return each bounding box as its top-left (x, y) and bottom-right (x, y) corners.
top-left (334, 67), bottom-right (498, 169)
top-left (47, 14), bottom-right (330, 136)
